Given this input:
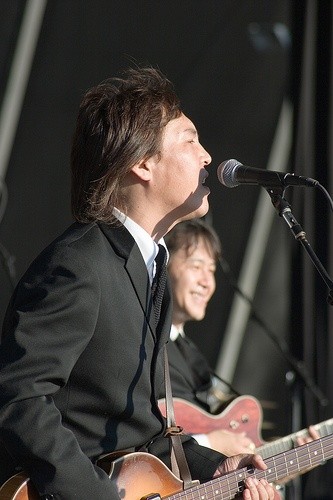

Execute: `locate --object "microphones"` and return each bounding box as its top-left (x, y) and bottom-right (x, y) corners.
top-left (216, 159), bottom-right (318, 190)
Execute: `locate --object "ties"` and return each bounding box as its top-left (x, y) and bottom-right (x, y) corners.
top-left (175, 335), bottom-right (202, 389)
top-left (153, 244), bottom-right (168, 332)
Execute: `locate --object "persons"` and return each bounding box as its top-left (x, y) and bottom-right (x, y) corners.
top-left (0, 65), bottom-right (276, 500)
top-left (164, 217), bottom-right (325, 487)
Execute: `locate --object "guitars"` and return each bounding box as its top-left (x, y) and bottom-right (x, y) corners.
top-left (0, 434), bottom-right (333, 500)
top-left (155, 393), bottom-right (332, 465)
